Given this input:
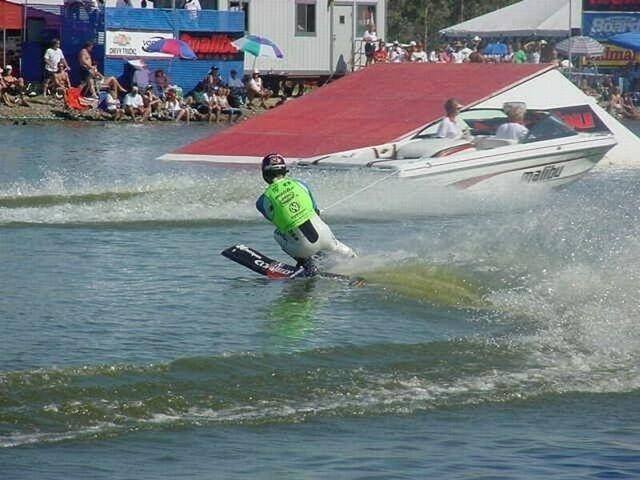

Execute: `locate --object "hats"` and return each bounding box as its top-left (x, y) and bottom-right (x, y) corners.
top-left (506, 107), bottom-right (527, 119)
top-left (525, 40), bottom-right (548, 50)
top-left (254, 70), bottom-right (260, 74)
top-left (6, 65), bottom-right (12, 70)
top-left (445, 99), bottom-right (462, 117)
top-left (454, 36), bottom-right (481, 46)
top-left (211, 65), bottom-right (219, 70)
top-left (409, 41), bottom-right (425, 46)
top-left (145, 85), bottom-right (152, 91)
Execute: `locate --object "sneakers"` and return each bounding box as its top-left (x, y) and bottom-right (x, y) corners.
top-left (305, 266), bottom-right (320, 278)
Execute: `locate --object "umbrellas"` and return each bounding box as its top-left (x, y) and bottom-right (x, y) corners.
top-left (554, 36), bottom-right (605, 85)
top-left (229, 34), bottom-right (284, 71)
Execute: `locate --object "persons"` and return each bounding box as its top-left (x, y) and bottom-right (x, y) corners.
top-left (495, 101), bottom-right (537, 141)
top-left (437, 97), bottom-right (472, 141)
top-left (551, 51), bottom-right (640, 121)
top-left (0, 38), bottom-right (274, 127)
top-left (364, 24), bottom-right (547, 64)
top-left (255, 153), bottom-right (359, 273)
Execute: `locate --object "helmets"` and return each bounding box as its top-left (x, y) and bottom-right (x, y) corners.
top-left (262, 153), bottom-right (286, 184)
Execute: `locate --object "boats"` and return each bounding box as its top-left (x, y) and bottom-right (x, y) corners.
top-left (291, 107), bottom-right (619, 209)
